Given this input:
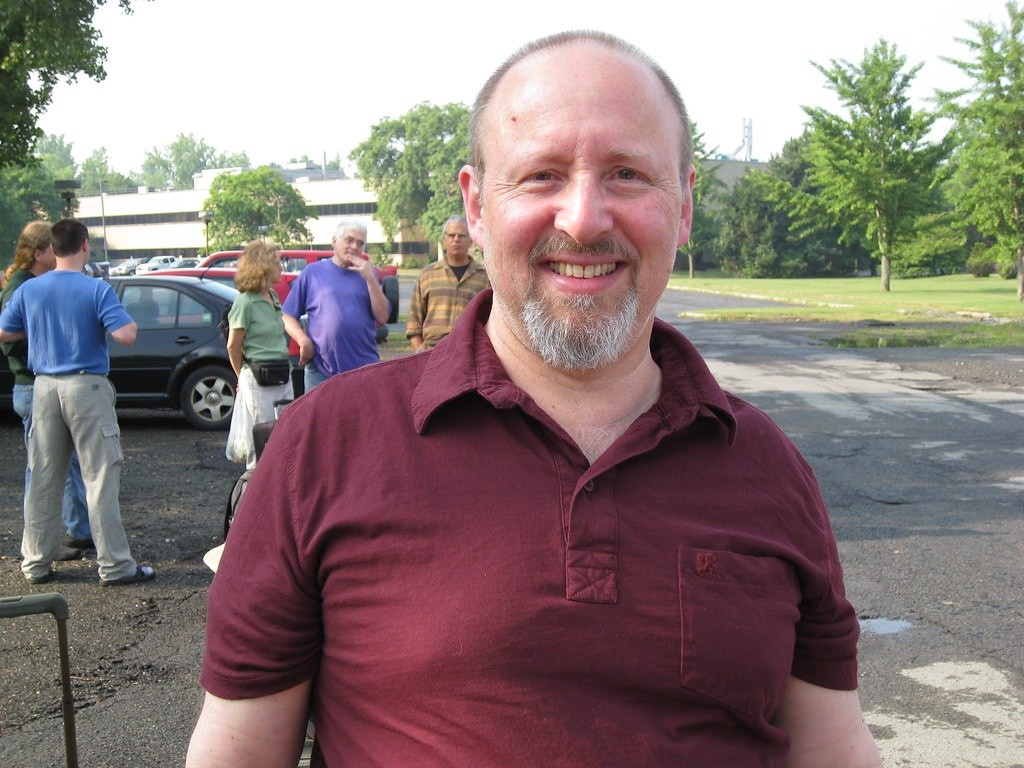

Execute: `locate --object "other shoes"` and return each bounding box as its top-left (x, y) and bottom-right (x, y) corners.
top-left (25, 565), bottom-right (53, 584)
top-left (99, 565), bottom-right (156, 586)
top-left (59, 545), bottom-right (82, 560)
top-left (61, 533), bottom-right (95, 548)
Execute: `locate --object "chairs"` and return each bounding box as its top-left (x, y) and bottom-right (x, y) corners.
top-left (126, 300), bottom-right (160, 327)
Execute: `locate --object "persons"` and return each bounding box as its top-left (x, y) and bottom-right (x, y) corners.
top-left (227, 238), bottom-right (294, 474)
top-left (186, 31), bottom-right (885, 768)
top-left (0, 216), bottom-right (155, 587)
top-left (405, 215), bottom-right (492, 356)
top-left (283, 217), bottom-right (391, 395)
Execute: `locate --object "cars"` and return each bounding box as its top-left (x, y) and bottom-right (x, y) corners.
top-left (104, 248), bottom-right (401, 348)
top-left (1, 274), bottom-right (249, 429)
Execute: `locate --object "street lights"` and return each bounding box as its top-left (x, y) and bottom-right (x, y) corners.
top-left (197, 209), bottom-right (215, 257)
top-left (55, 179), bottom-right (81, 218)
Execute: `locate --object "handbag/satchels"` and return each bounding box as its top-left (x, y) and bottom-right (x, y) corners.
top-left (225, 385), bottom-right (254, 464)
top-left (250, 358), bottom-right (290, 387)
